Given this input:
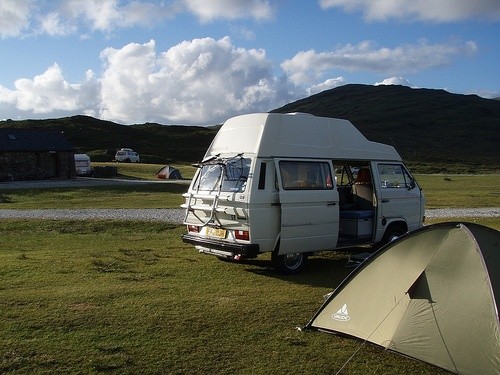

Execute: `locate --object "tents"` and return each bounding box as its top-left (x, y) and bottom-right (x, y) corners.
top-left (304, 221), bottom-right (500, 375)
top-left (154, 165), bottom-right (182, 180)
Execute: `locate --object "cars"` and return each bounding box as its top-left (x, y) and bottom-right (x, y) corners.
top-left (115, 148), bottom-right (140, 163)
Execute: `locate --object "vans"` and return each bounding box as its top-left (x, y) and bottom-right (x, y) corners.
top-left (74, 154), bottom-right (91, 177)
top-left (180, 112), bottom-right (426, 276)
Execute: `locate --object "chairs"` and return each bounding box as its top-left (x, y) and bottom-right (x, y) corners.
top-left (305, 167), bottom-right (319, 185)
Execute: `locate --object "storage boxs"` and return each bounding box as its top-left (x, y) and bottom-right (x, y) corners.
top-left (339, 208), bottom-right (372, 236)
